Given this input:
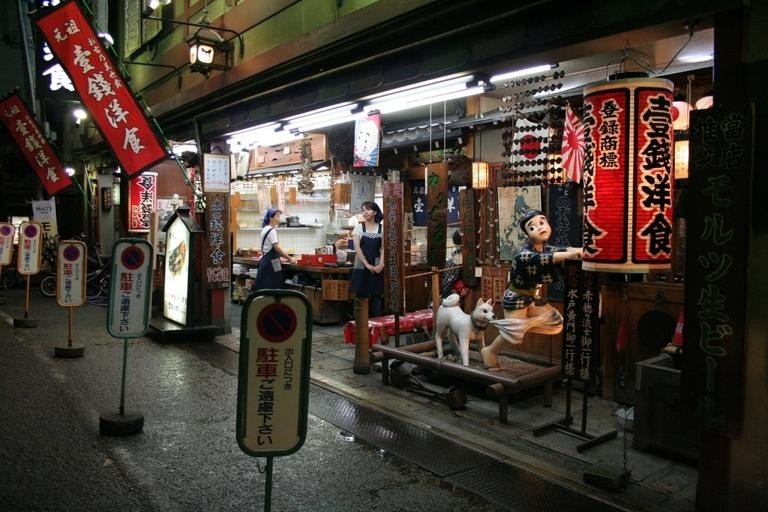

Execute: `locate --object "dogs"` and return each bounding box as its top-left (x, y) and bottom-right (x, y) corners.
top-left (434, 293), bottom-right (498, 367)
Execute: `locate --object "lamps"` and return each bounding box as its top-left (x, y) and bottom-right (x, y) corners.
top-left (184, 0), bottom-right (235, 81)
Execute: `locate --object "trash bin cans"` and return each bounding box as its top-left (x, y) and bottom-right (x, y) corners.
top-left (633, 354), bottom-right (684, 461)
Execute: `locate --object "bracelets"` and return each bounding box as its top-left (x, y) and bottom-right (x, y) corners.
top-left (378, 263), bottom-right (384, 268)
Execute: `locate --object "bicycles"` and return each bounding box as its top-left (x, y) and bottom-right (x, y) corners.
top-left (0, 231), bottom-right (113, 301)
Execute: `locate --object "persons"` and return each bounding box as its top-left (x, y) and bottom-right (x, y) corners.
top-left (349, 201), bottom-right (386, 316)
top-left (430, 230), bottom-right (464, 307)
top-left (504, 187), bottom-right (529, 239)
top-left (253, 208), bottom-right (296, 290)
top-left (480, 210), bottom-right (583, 372)
top-left (354, 121), bottom-right (378, 162)
